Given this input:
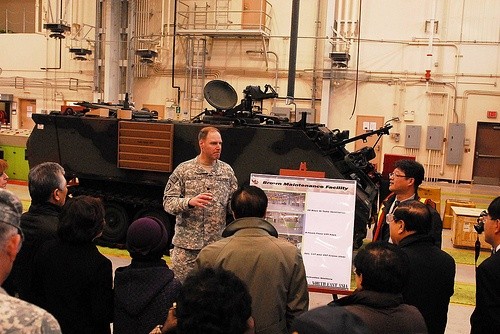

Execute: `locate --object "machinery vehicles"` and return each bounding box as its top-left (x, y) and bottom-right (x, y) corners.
top-left (23, 79), bottom-right (399, 256)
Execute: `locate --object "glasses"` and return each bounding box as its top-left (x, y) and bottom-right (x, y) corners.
top-left (389, 173), bottom-right (406, 178)
top-left (386, 214), bottom-right (397, 224)
top-left (482, 216), bottom-right (498, 223)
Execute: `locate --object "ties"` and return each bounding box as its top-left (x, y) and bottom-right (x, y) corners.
top-left (390, 200), bottom-right (400, 214)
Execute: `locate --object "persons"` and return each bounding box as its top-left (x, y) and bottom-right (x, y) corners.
top-left (470, 196), bottom-right (500, 334)
top-left (0, 127), bottom-right (456, 334)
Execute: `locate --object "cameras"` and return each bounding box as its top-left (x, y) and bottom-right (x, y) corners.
top-left (474, 210), bottom-right (488, 234)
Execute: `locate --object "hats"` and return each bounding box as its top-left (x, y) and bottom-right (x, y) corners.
top-left (0, 189), bottom-right (25, 242)
top-left (125, 216), bottom-right (168, 255)
